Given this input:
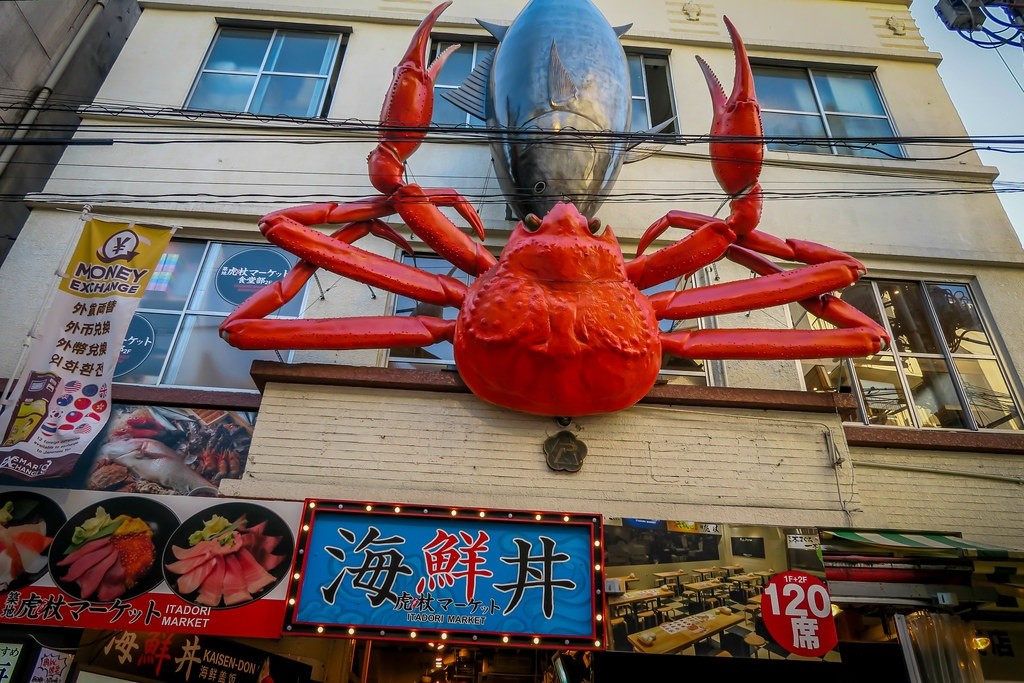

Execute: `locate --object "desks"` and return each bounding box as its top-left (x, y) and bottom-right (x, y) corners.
top-left (685, 581), bottom-right (726, 603)
top-left (652, 571), bottom-right (688, 594)
top-left (727, 575), bottom-right (761, 590)
top-left (720, 566), bottom-right (744, 577)
top-left (627, 607), bottom-right (744, 654)
top-left (692, 569), bottom-right (722, 580)
top-left (754, 572), bottom-right (775, 585)
top-left (747, 593), bottom-right (761, 604)
top-left (607, 576), bottom-right (640, 589)
top-left (607, 587), bottom-right (675, 632)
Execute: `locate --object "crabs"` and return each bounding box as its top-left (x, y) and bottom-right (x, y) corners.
top-left (219, 1), bottom-right (891, 416)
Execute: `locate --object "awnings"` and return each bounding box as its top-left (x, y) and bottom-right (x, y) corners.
top-left (824, 530), bottom-right (1024, 578)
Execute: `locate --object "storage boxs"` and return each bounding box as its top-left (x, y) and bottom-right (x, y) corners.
top-left (605, 579), bottom-right (626, 592)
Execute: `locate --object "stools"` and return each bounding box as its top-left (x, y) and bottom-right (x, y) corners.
top-left (610, 570), bottom-right (771, 660)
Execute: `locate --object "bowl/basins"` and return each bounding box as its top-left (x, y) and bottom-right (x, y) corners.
top-left (48, 497), bottom-right (182, 605)
top-left (0, 491), bottom-right (68, 593)
top-left (162, 502), bottom-right (294, 610)
